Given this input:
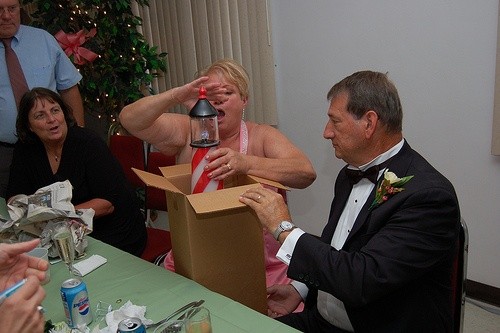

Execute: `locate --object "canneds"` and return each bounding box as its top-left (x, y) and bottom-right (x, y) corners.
top-left (116, 317), bottom-right (147, 333)
top-left (61, 278), bottom-right (92, 329)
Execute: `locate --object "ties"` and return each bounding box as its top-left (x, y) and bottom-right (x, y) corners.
top-left (1, 37), bottom-right (29, 113)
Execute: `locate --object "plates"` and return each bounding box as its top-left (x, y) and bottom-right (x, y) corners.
top-left (153, 320), bottom-right (186, 333)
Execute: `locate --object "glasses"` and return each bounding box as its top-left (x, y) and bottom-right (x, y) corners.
top-left (0, 1), bottom-right (23, 16)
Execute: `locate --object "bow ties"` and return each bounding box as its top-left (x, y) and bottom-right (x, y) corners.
top-left (345, 157), bottom-right (393, 185)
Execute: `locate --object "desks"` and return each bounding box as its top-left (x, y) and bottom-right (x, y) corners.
top-left (0, 195), bottom-right (303, 333)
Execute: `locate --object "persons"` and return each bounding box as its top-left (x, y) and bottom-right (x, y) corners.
top-left (118, 58), bottom-right (318, 313)
top-left (235, 71), bottom-right (461, 333)
top-left (0, 0), bottom-right (85, 198)
top-left (5, 85), bottom-right (148, 257)
top-left (0, 238), bottom-right (48, 333)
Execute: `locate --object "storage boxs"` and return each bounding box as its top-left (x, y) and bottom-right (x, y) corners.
top-left (130, 162), bottom-right (291, 318)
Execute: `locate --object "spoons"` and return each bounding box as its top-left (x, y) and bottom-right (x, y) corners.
top-left (164, 299), bottom-right (204, 333)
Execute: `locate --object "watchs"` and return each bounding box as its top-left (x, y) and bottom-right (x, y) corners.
top-left (273, 221), bottom-right (297, 240)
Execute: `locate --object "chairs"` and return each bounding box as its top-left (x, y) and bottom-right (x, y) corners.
top-left (108, 120), bottom-right (176, 267)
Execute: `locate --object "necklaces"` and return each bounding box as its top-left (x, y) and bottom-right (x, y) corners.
top-left (54, 151), bottom-right (58, 162)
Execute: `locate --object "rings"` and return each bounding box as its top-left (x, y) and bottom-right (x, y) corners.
top-left (37, 305), bottom-right (44, 313)
top-left (227, 164), bottom-right (231, 170)
top-left (257, 195), bottom-right (261, 199)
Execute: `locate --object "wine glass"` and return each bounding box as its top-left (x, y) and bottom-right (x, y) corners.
top-left (51, 222), bottom-right (75, 279)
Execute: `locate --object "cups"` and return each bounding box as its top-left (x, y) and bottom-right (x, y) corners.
top-left (26, 248), bottom-right (50, 285)
top-left (185, 307), bottom-right (211, 333)
